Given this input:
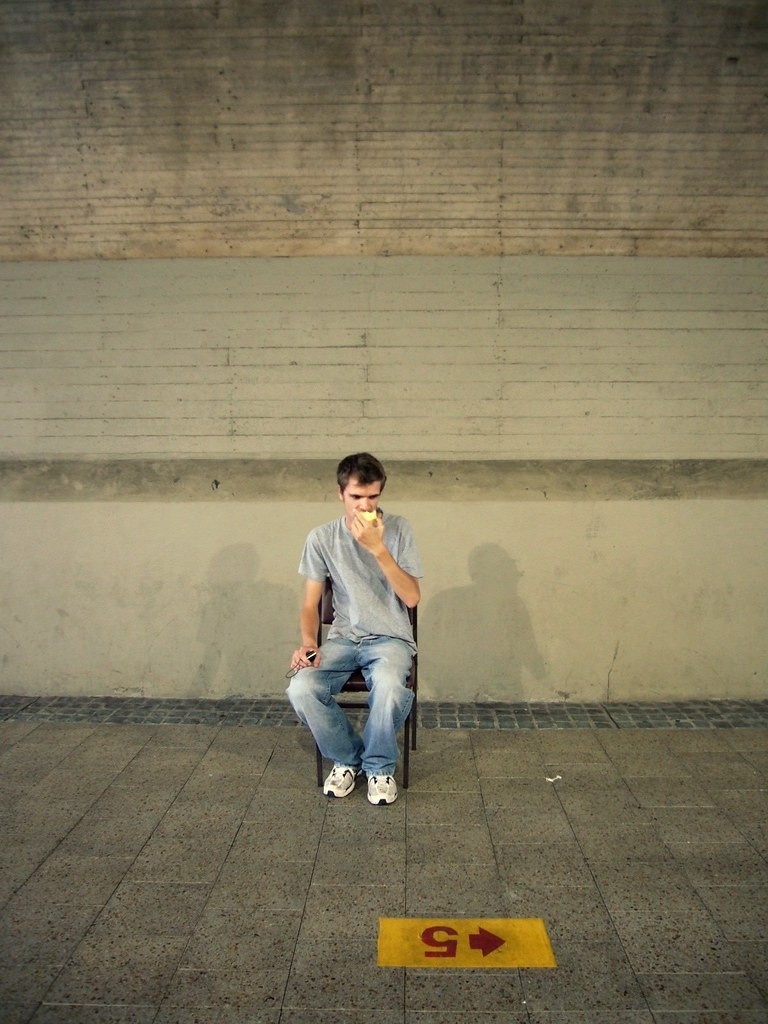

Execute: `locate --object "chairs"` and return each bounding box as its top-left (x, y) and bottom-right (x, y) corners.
top-left (315, 576), bottom-right (419, 790)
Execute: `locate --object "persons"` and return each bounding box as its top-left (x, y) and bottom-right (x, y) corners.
top-left (285, 453), bottom-right (424, 805)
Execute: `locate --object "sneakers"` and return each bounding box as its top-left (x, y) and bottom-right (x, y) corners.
top-left (323, 764), bottom-right (363, 798)
top-left (367, 775), bottom-right (397, 805)
